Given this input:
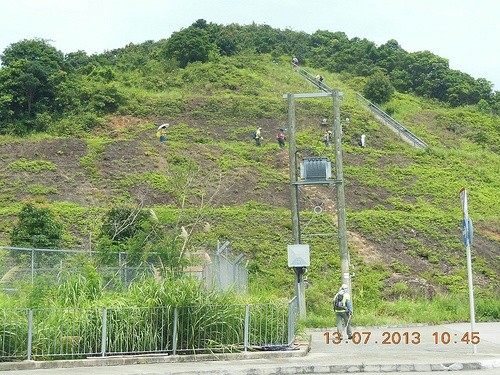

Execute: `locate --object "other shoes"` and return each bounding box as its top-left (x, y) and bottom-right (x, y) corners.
top-left (340, 339), bottom-right (343, 340)
top-left (348, 336), bottom-right (354, 339)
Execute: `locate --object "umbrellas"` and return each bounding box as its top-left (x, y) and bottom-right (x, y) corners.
top-left (157, 124), bottom-right (169, 130)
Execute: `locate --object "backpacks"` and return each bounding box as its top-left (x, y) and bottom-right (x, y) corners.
top-left (275, 134), bottom-right (279, 140)
top-left (156, 128), bottom-right (162, 138)
top-left (252, 132), bottom-right (256, 139)
top-left (323, 135), bottom-right (327, 142)
top-left (334, 291), bottom-right (347, 310)
top-left (294, 59), bottom-right (298, 63)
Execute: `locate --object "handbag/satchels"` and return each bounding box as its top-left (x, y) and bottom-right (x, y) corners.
top-left (358, 141), bottom-right (362, 146)
top-left (320, 76), bottom-right (323, 81)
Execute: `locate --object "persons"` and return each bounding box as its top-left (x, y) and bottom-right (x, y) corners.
top-left (324, 130), bottom-right (332, 148)
top-left (276, 129), bottom-right (286, 147)
top-left (332, 284), bottom-right (352, 339)
top-left (316, 74), bottom-right (323, 89)
top-left (254, 127), bottom-right (264, 148)
top-left (159, 126), bottom-right (166, 143)
top-left (290, 54), bottom-right (299, 71)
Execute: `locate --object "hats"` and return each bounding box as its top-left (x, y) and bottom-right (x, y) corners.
top-left (259, 127), bottom-right (262, 130)
top-left (280, 129), bottom-right (284, 131)
top-left (162, 126), bottom-right (166, 128)
top-left (340, 284), bottom-right (347, 291)
